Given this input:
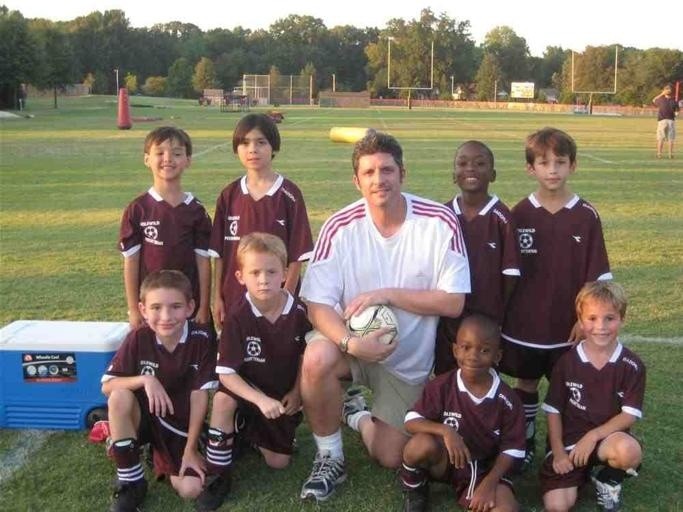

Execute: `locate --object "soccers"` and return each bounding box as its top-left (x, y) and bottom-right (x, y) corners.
top-left (345, 305), bottom-right (400, 345)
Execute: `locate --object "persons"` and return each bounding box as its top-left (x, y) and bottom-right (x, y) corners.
top-left (99, 267), bottom-right (221, 510)
top-left (394, 314), bottom-right (526, 511)
top-left (300, 131), bottom-right (471, 503)
top-left (120, 125), bottom-right (211, 330)
top-left (432, 140), bottom-right (523, 390)
top-left (204, 114), bottom-right (315, 332)
top-left (509, 127), bottom-right (614, 474)
top-left (539, 282), bottom-right (646, 512)
top-left (195, 231), bottom-right (308, 510)
top-left (652, 83), bottom-right (679, 161)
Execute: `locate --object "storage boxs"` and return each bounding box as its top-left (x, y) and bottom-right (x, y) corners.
top-left (0, 319), bottom-right (130, 431)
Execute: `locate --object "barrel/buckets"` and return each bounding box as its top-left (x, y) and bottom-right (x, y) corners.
top-left (328, 126), bottom-right (370, 143)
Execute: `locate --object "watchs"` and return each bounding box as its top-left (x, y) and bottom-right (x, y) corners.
top-left (339, 332), bottom-right (354, 354)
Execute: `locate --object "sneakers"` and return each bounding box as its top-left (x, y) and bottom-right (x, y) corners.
top-left (401, 483), bottom-right (429, 512)
top-left (194, 460), bottom-right (233, 512)
top-left (110, 475), bottom-right (149, 512)
top-left (656, 152), bottom-right (674, 160)
top-left (590, 477), bottom-right (623, 511)
top-left (338, 383), bottom-right (376, 426)
top-left (519, 438), bottom-right (536, 473)
top-left (299, 449), bottom-right (349, 503)
